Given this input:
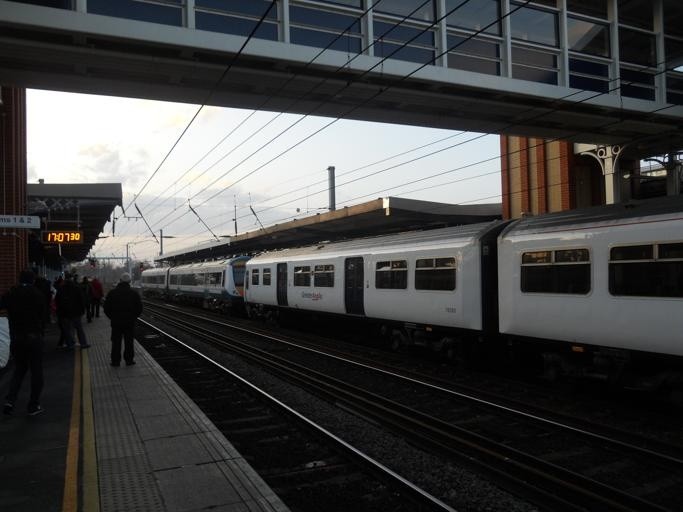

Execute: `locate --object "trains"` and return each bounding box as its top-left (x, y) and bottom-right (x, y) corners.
top-left (136, 198), bottom-right (683, 370)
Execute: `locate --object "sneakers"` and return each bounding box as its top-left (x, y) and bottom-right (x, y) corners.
top-left (25, 404), bottom-right (45, 416)
top-left (125, 359), bottom-right (137, 366)
top-left (1, 402), bottom-right (16, 415)
top-left (87, 320), bottom-right (92, 323)
top-left (95, 315), bottom-right (99, 317)
top-left (79, 343), bottom-right (91, 348)
top-left (56, 343), bottom-right (70, 349)
top-left (108, 361), bottom-right (120, 368)
top-left (91, 314), bottom-right (94, 317)
top-left (72, 342), bottom-right (81, 346)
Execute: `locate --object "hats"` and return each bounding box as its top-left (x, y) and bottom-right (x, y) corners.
top-left (119, 272), bottom-right (131, 282)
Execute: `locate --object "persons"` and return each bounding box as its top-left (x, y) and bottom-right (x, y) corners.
top-left (0, 272), bottom-right (48, 417)
top-left (103, 273), bottom-right (142, 366)
top-left (0, 266), bottom-right (103, 372)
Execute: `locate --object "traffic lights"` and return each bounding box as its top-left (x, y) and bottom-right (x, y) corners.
top-left (88, 259), bottom-right (96, 269)
